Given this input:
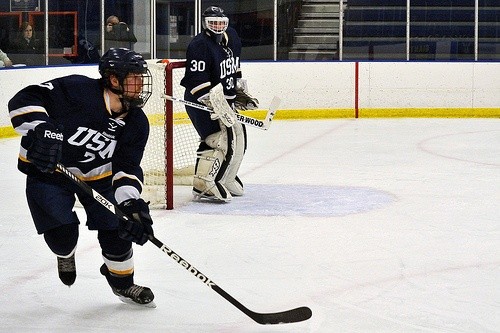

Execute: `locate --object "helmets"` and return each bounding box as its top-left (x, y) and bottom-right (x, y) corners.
top-left (98, 47), bottom-right (152, 113)
top-left (202, 6), bottom-right (229, 34)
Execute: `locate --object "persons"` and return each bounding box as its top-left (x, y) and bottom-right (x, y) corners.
top-left (62, 31), bottom-right (101, 64)
top-left (0, 49), bottom-right (12, 67)
top-left (106, 16), bottom-right (137, 50)
top-left (7, 47), bottom-right (155, 305)
top-left (179, 6), bottom-right (260, 203)
top-left (12, 22), bottom-right (46, 65)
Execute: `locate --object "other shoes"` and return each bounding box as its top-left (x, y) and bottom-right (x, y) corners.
top-left (57, 252), bottom-right (76, 288)
top-left (100, 263), bottom-right (157, 308)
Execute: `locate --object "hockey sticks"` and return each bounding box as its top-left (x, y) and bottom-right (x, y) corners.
top-left (159, 92), bottom-right (281, 131)
top-left (57, 164), bottom-right (313, 326)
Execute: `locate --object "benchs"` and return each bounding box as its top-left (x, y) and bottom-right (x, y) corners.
top-left (340, 0), bottom-right (499, 61)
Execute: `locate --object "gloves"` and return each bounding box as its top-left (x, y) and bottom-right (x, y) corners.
top-left (26, 122), bottom-right (63, 174)
top-left (116, 199), bottom-right (154, 246)
